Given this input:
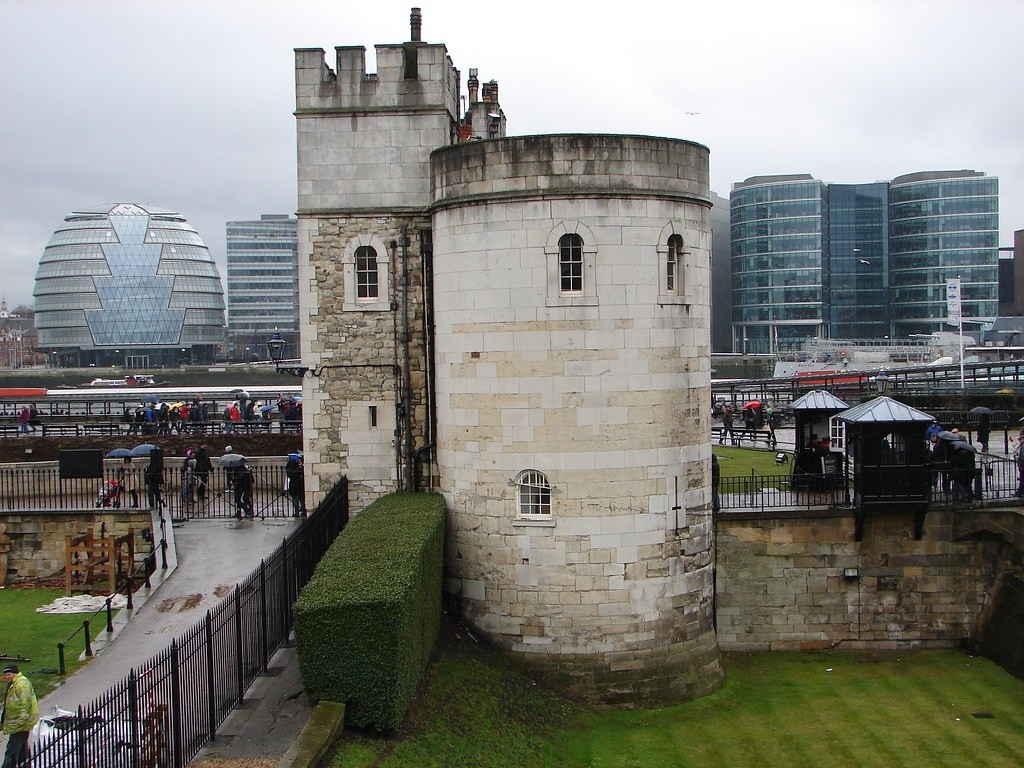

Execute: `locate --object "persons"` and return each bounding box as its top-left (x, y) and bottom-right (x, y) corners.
top-left (124, 393), bottom-right (302, 434)
top-left (20, 404), bottom-right (37, 434)
top-left (0, 662), bottom-right (39, 768)
top-left (96, 444), bottom-right (305, 517)
top-left (977, 412), bottom-right (990, 452)
top-left (0, 409), bottom-right (130, 416)
top-left (925, 420), bottom-right (977, 502)
top-left (1010, 421), bottom-right (1024, 498)
top-left (806, 434), bottom-right (831, 493)
top-left (712, 401), bottom-right (777, 445)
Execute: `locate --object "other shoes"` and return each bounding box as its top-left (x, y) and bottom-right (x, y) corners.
top-left (292, 514), bottom-right (299, 517)
top-left (200, 496), bottom-right (208, 499)
top-left (301, 513), bottom-right (307, 515)
top-left (132, 505), bottom-right (138, 508)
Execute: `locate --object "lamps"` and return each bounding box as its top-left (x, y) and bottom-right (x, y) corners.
top-left (776, 452), bottom-right (789, 466)
top-left (843, 567), bottom-right (858, 581)
top-left (266, 326), bottom-right (309, 379)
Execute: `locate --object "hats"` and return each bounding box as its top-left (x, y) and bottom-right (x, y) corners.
top-left (813, 434), bottom-right (819, 439)
top-left (822, 435), bottom-right (830, 441)
top-left (4, 665), bottom-right (19, 674)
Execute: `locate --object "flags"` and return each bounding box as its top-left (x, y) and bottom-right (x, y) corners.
top-left (948, 279), bottom-right (961, 326)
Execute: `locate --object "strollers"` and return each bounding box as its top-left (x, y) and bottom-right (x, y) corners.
top-left (95, 474), bottom-right (125, 508)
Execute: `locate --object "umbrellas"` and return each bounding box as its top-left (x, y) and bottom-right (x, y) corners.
top-left (154, 402), bottom-right (171, 410)
top-left (260, 405), bottom-right (275, 411)
top-left (970, 406), bottom-right (993, 414)
top-left (168, 402), bottom-right (183, 413)
top-left (743, 402), bottom-right (760, 409)
top-left (1018, 416), bottom-right (1024, 421)
top-left (131, 444), bottom-right (160, 457)
top-left (140, 395), bottom-right (162, 404)
top-left (938, 430), bottom-right (961, 440)
top-left (231, 389), bottom-right (250, 399)
top-left (287, 454), bottom-right (302, 463)
top-left (279, 396), bottom-right (291, 401)
top-left (218, 454), bottom-right (249, 469)
top-left (293, 397), bottom-right (303, 400)
top-left (106, 448), bottom-right (132, 457)
top-left (949, 441), bottom-right (977, 453)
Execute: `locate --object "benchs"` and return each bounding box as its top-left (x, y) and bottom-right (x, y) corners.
top-left (83, 423), bottom-right (123, 435)
top-left (184, 422), bottom-right (221, 434)
top-left (232, 420), bottom-right (272, 433)
top-left (42, 423), bottom-right (82, 436)
top-left (0, 424), bottom-right (19, 437)
top-left (279, 419), bottom-right (303, 433)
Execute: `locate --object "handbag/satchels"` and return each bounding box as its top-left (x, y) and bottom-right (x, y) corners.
top-left (762, 422), bottom-right (771, 434)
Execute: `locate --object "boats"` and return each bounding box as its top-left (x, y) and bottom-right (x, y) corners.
top-left (55, 375), bottom-right (171, 389)
